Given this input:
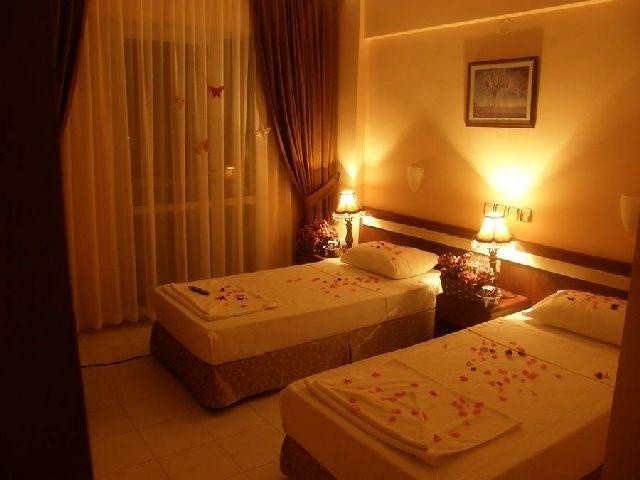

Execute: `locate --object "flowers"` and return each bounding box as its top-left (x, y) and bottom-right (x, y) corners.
top-left (296, 217), bottom-right (339, 254)
top-left (432, 249), bottom-right (496, 302)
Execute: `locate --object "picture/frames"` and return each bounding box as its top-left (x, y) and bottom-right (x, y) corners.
top-left (465, 56), bottom-right (538, 128)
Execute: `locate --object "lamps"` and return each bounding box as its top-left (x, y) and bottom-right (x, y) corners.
top-left (476, 213), bottom-right (514, 275)
top-left (331, 191), bottom-right (364, 248)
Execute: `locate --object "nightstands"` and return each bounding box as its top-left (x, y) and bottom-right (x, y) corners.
top-left (295, 248), bottom-right (330, 264)
top-left (434, 285), bottom-right (530, 339)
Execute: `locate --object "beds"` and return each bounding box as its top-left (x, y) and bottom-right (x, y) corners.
top-left (149, 239), bottom-right (445, 410)
top-left (279, 288), bottom-right (628, 480)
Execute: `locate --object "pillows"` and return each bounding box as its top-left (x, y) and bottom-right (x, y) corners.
top-left (341, 240), bottom-right (440, 278)
top-left (521, 289), bottom-right (627, 346)
